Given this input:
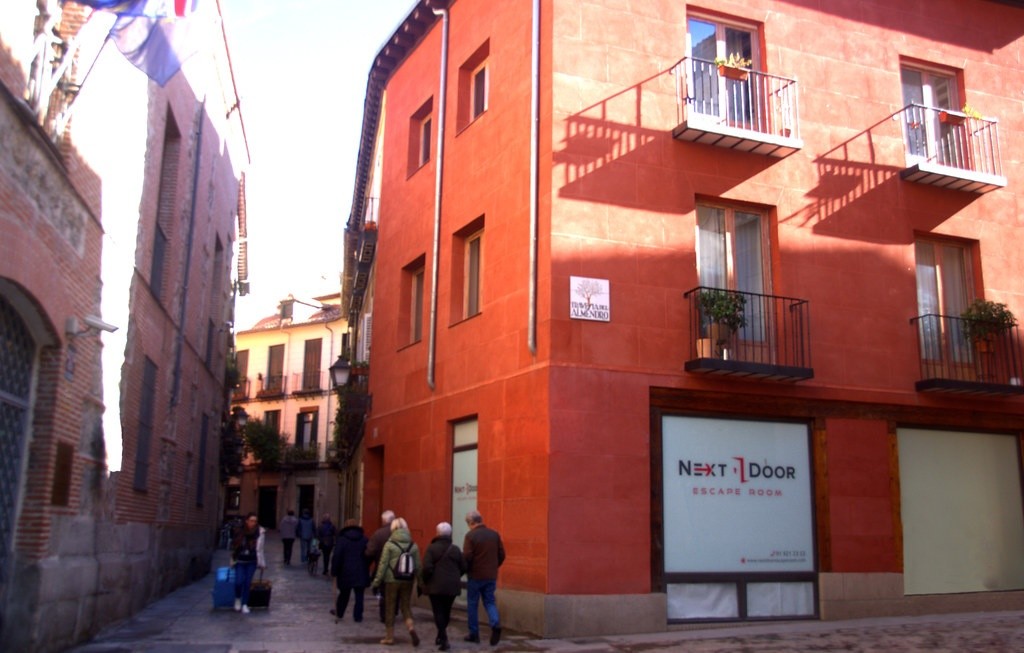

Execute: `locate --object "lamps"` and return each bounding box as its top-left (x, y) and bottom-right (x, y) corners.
top-left (233, 406), bottom-right (249, 425)
top-left (329, 356), bottom-right (351, 388)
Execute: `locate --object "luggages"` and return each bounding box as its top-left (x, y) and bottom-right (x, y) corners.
top-left (211, 565), bottom-right (236, 608)
top-left (247, 566), bottom-right (272, 607)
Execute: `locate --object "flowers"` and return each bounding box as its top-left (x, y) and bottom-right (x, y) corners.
top-left (714, 52), bottom-right (751, 69)
top-left (961, 102), bottom-right (984, 119)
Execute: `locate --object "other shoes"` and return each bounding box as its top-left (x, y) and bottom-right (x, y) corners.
top-left (330, 609), bottom-right (337, 616)
top-left (435, 639), bottom-right (450, 650)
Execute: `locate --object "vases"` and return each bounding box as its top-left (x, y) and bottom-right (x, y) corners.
top-left (939, 110), bottom-right (967, 126)
top-left (696, 339), bottom-right (718, 358)
top-left (717, 64), bottom-right (748, 81)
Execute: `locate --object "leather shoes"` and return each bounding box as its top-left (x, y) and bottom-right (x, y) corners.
top-left (490, 626), bottom-right (502, 645)
top-left (381, 638), bottom-right (394, 644)
top-left (464, 634), bottom-right (480, 642)
top-left (409, 628), bottom-right (420, 646)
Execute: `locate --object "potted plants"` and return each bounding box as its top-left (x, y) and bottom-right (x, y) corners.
top-left (959, 298), bottom-right (1018, 353)
top-left (696, 289), bottom-right (749, 345)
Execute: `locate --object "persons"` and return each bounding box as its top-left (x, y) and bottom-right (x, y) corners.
top-left (228, 512), bottom-right (266, 615)
top-left (369, 516), bottom-right (420, 647)
top-left (222, 514), bottom-right (234, 552)
top-left (327, 515), bottom-right (372, 622)
top-left (306, 537), bottom-right (322, 576)
top-left (366, 510), bottom-right (402, 623)
top-left (278, 508), bottom-right (298, 567)
top-left (418, 522), bottom-right (466, 650)
top-left (461, 510), bottom-right (507, 647)
top-left (230, 517), bottom-right (246, 552)
top-left (295, 509), bottom-right (318, 563)
top-left (314, 515), bottom-right (336, 575)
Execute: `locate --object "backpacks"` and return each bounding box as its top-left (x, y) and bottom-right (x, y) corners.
top-left (388, 540), bottom-right (415, 580)
